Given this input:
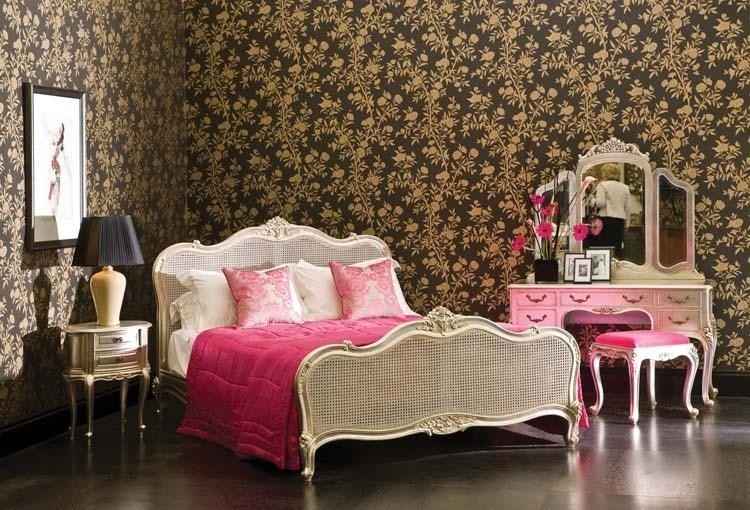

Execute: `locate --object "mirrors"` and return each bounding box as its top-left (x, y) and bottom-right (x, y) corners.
top-left (577, 136), bottom-right (652, 273)
top-left (652, 166), bottom-right (698, 274)
top-left (534, 169), bottom-right (580, 263)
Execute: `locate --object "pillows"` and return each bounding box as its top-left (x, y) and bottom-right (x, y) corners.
top-left (168, 255), bottom-right (420, 333)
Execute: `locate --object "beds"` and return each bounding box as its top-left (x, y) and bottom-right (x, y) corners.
top-left (150, 217), bottom-right (590, 483)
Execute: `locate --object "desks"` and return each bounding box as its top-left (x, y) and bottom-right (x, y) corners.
top-left (506, 278), bottom-right (718, 408)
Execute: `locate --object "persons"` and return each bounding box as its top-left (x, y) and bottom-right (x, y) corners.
top-left (589, 164), bottom-right (631, 261)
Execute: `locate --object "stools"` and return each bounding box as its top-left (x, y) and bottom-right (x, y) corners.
top-left (587, 328), bottom-right (701, 424)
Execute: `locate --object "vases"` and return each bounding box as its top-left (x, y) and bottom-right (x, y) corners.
top-left (532, 260), bottom-right (561, 284)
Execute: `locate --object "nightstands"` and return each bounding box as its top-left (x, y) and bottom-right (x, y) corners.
top-left (66, 319), bottom-right (153, 438)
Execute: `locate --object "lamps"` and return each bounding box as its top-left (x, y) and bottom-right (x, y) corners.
top-left (70, 215), bottom-right (148, 327)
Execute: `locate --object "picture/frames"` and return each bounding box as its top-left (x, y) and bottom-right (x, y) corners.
top-left (586, 247), bottom-right (612, 283)
top-left (20, 82), bottom-right (90, 252)
top-left (564, 252), bottom-right (582, 281)
top-left (575, 259), bottom-right (592, 285)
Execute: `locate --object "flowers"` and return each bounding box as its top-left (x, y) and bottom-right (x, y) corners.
top-left (510, 161), bottom-right (603, 259)
